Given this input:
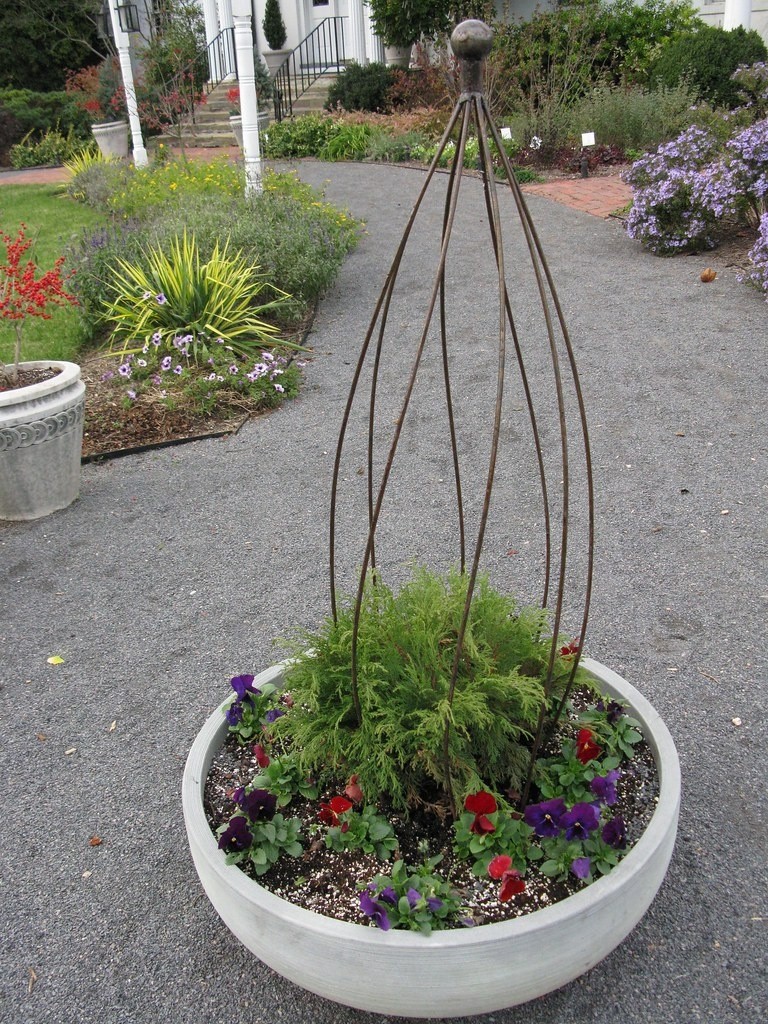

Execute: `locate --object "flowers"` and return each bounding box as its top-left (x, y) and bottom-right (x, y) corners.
top-left (75, 88), bottom-right (125, 124)
top-left (0, 221), bottom-right (82, 380)
top-left (228, 563), bottom-right (629, 917)
top-left (225, 89), bottom-right (263, 115)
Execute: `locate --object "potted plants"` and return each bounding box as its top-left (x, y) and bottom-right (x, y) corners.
top-left (262, 0), bottom-right (294, 76)
top-left (363, 0), bottom-right (420, 68)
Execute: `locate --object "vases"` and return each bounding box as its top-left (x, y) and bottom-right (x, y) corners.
top-left (229, 112), bottom-right (270, 154)
top-left (181, 642), bottom-right (683, 1014)
top-left (91, 120), bottom-right (128, 163)
top-left (0, 361), bottom-right (87, 521)
